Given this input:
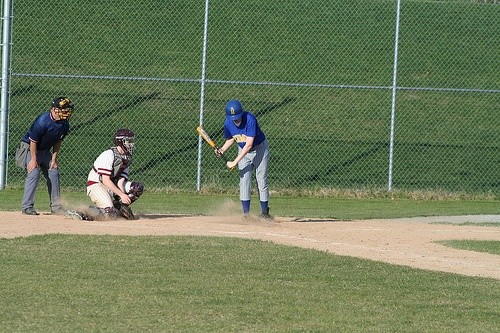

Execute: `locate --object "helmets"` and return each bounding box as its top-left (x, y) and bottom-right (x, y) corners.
top-left (226, 100), bottom-right (243, 120)
top-left (51, 97), bottom-right (74, 110)
top-left (114, 129), bottom-right (134, 146)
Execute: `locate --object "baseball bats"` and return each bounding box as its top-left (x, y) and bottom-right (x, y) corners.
top-left (196, 126), bottom-right (228, 162)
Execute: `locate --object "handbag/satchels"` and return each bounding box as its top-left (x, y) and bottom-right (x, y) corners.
top-left (16, 140), bottom-right (30, 170)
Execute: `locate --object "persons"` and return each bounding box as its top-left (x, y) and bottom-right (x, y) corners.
top-left (67, 129), bottom-right (144, 221)
top-left (214, 99), bottom-right (271, 221)
top-left (20, 96), bottom-right (74, 215)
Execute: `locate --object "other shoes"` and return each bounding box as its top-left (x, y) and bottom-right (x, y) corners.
top-left (51, 207), bottom-right (66, 215)
top-left (22, 208), bottom-right (37, 215)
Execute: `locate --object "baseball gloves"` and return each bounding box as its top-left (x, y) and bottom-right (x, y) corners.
top-left (119, 200), bottom-right (135, 220)
